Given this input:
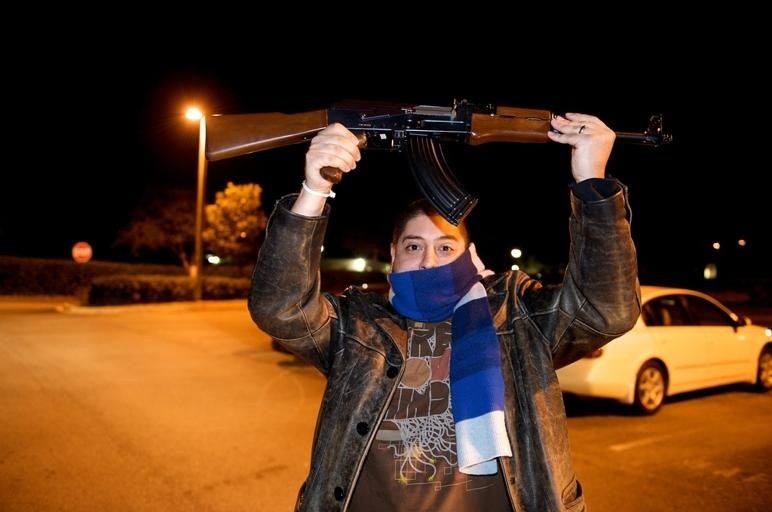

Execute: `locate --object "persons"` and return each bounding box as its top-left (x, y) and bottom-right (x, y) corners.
top-left (248, 113), bottom-right (641, 512)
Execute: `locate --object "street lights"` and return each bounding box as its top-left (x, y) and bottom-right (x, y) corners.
top-left (183, 105), bottom-right (207, 301)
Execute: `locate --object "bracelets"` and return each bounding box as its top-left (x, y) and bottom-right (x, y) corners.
top-left (303, 178), bottom-right (335, 199)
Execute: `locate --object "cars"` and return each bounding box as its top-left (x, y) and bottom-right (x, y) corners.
top-left (555, 285), bottom-right (772, 416)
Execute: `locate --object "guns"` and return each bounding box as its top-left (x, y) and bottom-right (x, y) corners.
top-left (206, 99), bottom-right (673, 225)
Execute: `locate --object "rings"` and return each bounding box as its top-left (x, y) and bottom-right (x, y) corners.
top-left (579, 126), bottom-right (585, 134)
